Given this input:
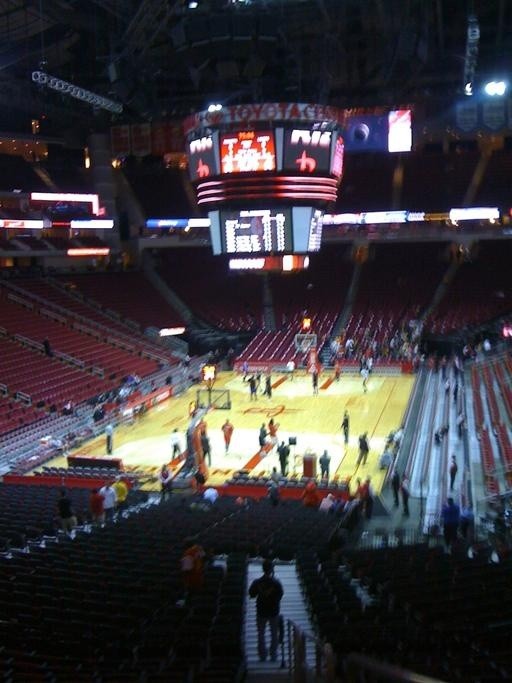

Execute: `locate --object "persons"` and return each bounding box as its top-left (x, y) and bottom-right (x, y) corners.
top-left (179, 546), bottom-right (213, 588)
top-left (428, 313), bottom-right (512, 567)
top-left (57, 476), bottom-right (128, 531)
top-left (157, 400), bottom-right (410, 520)
top-left (105, 422), bottom-right (113, 455)
top-left (240, 332), bottom-right (428, 400)
top-left (249, 560), bottom-right (284, 652)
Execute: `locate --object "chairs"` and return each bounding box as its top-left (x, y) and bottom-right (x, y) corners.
top-left (0, 262), bottom-right (512, 682)
